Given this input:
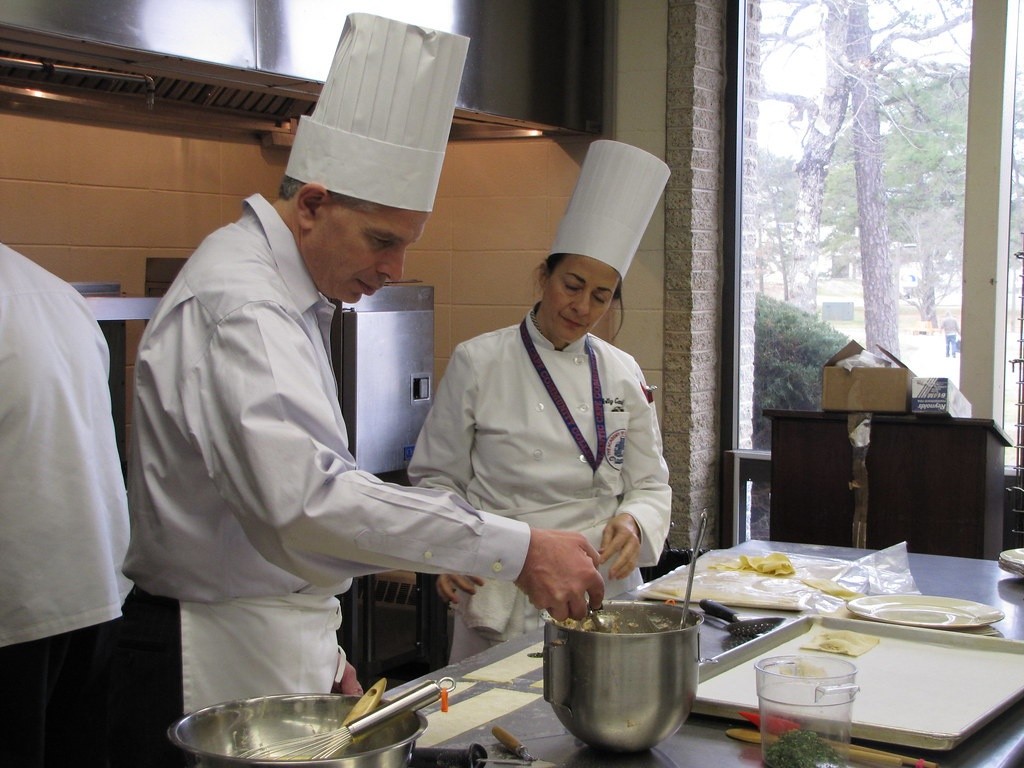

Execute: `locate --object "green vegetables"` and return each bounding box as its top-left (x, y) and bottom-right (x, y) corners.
top-left (764, 728), bottom-right (846, 768)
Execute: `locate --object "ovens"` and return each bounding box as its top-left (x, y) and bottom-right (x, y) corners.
top-left (323, 286), bottom-right (438, 478)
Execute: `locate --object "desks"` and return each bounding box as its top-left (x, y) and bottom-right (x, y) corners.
top-left (383, 537), bottom-right (1024, 768)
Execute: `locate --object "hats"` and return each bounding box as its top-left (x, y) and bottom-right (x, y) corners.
top-left (544, 138), bottom-right (671, 282)
top-left (285, 13), bottom-right (471, 210)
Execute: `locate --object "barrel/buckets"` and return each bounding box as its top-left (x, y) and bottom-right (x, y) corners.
top-left (541, 599), bottom-right (703, 754)
top-left (754, 654), bottom-right (861, 768)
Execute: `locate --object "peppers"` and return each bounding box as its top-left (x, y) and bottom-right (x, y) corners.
top-left (739, 711), bottom-right (802, 736)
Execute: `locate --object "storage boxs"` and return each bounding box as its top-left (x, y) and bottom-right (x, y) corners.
top-left (910, 378), bottom-right (971, 418)
top-left (822, 340), bottom-right (913, 413)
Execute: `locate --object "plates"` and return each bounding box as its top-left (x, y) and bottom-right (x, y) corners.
top-left (847, 595), bottom-right (1005, 627)
top-left (998, 548), bottom-right (1024, 578)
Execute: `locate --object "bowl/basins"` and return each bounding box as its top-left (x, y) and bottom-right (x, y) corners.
top-left (166, 693), bottom-right (429, 768)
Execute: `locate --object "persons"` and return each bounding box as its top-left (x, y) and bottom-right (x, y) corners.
top-left (407, 140), bottom-right (672, 663)
top-left (108, 13), bottom-right (605, 768)
top-left (0, 241), bottom-right (137, 768)
